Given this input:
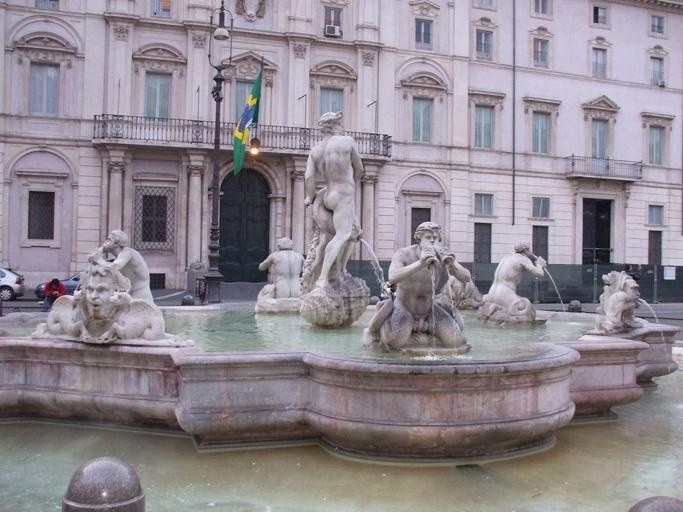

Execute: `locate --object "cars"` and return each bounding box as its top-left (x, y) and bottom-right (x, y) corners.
top-left (0, 269), bottom-right (81, 301)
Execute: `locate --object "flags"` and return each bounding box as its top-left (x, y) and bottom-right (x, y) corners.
top-left (232, 71), bottom-right (261, 176)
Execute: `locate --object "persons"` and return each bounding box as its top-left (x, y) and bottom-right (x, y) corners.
top-left (88, 229), bottom-right (154, 304)
top-left (485, 239), bottom-right (546, 312)
top-left (303, 110), bottom-right (366, 295)
top-left (376, 221), bottom-right (470, 348)
top-left (257, 236), bottom-right (305, 298)
top-left (600, 271), bottom-right (640, 322)
top-left (69, 260), bottom-right (128, 338)
top-left (41, 279), bottom-right (66, 311)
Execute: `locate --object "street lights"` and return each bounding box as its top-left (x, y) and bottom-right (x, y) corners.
top-left (202, 0), bottom-right (234, 303)
